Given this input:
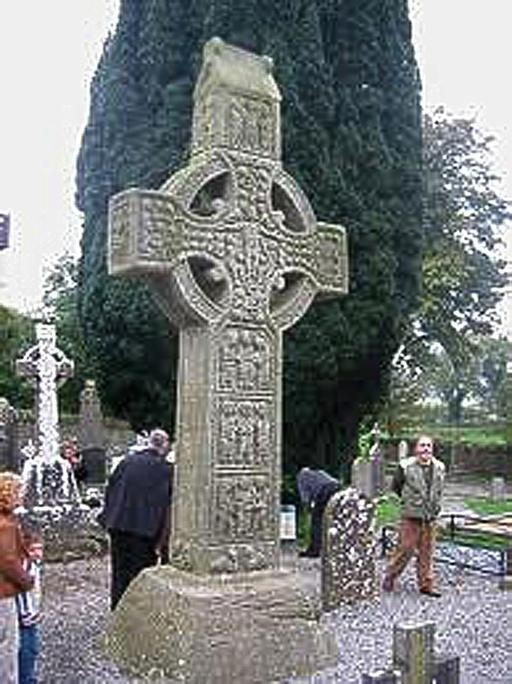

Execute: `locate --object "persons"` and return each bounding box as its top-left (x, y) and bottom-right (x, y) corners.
top-left (60, 445), bottom-right (87, 487)
top-left (14, 524), bottom-right (45, 684)
top-left (296, 466), bottom-right (344, 557)
top-left (0, 471), bottom-right (37, 684)
top-left (379, 434), bottom-right (444, 597)
top-left (96, 429), bottom-right (174, 612)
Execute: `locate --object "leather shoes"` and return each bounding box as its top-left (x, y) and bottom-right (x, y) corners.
top-left (382, 574), bottom-right (442, 598)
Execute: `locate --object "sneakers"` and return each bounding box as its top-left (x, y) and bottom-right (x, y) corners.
top-left (299, 549), bottom-right (322, 558)
